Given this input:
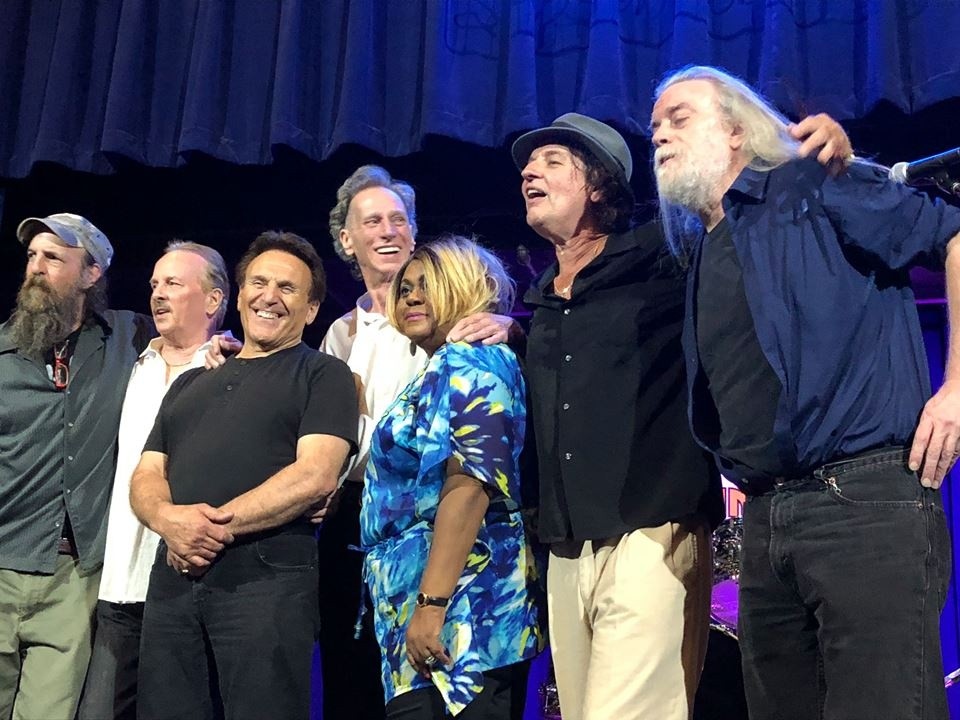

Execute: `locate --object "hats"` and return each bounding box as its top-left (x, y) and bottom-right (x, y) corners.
top-left (511, 111), bottom-right (635, 194)
top-left (18, 212), bottom-right (113, 273)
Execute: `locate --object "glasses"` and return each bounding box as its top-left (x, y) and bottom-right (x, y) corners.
top-left (54, 340), bottom-right (69, 388)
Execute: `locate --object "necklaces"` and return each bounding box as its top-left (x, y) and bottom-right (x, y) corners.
top-left (163, 359), bottom-right (194, 369)
top-left (554, 237), bottom-right (606, 295)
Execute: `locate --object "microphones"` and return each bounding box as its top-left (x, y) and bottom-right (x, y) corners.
top-left (887, 147), bottom-right (959, 187)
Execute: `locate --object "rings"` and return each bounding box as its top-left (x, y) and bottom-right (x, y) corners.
top-left (424, 656), bottom-right (436, 666)
top-left (181, 568), bottom-right (190, 574)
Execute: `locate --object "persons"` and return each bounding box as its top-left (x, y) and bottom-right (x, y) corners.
top-left (512, 111), bottom-right (855, 720)
top-left (648, 64), bottom-right (960, 720)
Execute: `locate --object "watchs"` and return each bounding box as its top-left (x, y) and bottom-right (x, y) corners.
top-left (416, 591), bottom-right (449, 608)
top-left (0, 162), bottom-right (545, 720)
